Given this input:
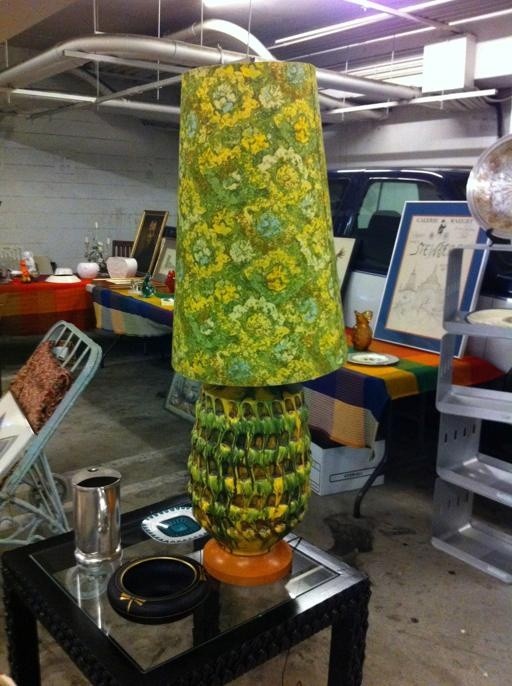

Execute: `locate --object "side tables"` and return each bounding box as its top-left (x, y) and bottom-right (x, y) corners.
top-left (1, 490), bottom-right (372, 685)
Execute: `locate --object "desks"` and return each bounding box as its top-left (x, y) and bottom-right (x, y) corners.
top-left (0, 272), bottom-right (163, 354)
top-left (84, 282), bottom-right (504, 518)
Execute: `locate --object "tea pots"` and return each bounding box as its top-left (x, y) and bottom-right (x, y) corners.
top-left (351, 310), bottom-right (373, 352)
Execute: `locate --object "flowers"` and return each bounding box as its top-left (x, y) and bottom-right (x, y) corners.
top-left (83, 220), bottom-right (112, 270)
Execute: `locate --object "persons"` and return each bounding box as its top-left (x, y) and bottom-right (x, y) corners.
top-left (136, 218), bottom-right (158, 271)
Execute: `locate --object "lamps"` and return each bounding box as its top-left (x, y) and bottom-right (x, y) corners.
top-left (170, 60), bottom-right (349, 589)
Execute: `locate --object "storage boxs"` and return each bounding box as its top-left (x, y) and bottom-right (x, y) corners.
top-left (310, 435), bottom-right (386, 496)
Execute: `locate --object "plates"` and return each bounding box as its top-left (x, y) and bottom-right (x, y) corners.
top-left (347, 351), bottom-right (400, 366)
top-left (465, 308), bottom-right (511, 327)
top-left (139, 503), bottom-right (210, 546)
top-left (45, 275), bottom-right (81, 284)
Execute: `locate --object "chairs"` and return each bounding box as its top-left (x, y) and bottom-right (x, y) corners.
top-left (358, 209), bottom-right (401, 264)
top-left (0, 320), bottom-right (104, 554)
top-left (111, 239), bottom-right (133, 258)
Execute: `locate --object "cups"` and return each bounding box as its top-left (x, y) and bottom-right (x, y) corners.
top-left (72, 466), bottom-right (123, 566)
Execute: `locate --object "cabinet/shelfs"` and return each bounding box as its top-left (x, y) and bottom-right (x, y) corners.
top-left (430, 242), bottom-right (512, 586)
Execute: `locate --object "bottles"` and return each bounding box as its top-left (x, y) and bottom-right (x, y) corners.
top-left (167, 270), bottom-right (175, 292)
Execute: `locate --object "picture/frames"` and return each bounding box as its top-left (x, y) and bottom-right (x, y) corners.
top-left (335, 235), bottom-right (359, 305)
top-left (129, 209), bottom-right (168, 277)
top-left (150, 237), bottom-right (177, 285)
top-left (371, 200), bottom-right (492, 360)
